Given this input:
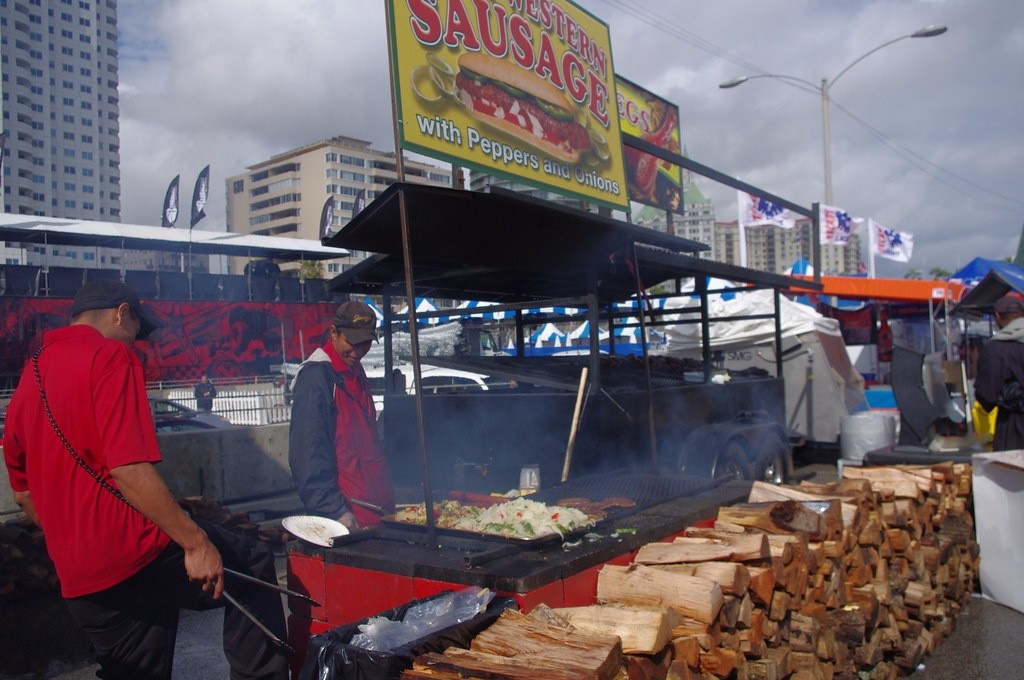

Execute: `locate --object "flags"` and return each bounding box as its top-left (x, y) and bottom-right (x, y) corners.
top-left (819, 204), bottom-right (864, 248)
top-left (871, 222), bottom-right (914, 263)
top-left (741, 189), bottom-right (796, 228)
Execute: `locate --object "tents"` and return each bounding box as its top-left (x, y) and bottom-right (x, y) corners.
top-left (364, 259), bottom-right (866, 348)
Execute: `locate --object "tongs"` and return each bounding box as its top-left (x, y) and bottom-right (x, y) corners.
top-left (213, 566), bottom-right (322, 657)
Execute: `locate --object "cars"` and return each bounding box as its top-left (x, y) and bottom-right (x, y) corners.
top-left (273, 360), bottom-right (496, 412)
top-left (147, 396), bottom-right (235, 435)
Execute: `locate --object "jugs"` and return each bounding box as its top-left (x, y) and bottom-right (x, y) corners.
top-left (519, 465), bottom-right (541, 492)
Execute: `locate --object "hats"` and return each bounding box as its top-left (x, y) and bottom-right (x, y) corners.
top-left (70, 279), bottom-right (167, 340)
top-left (333, 301), bottom-right (379, 345)
top-left (994, 297), bottom-right (1024, 312)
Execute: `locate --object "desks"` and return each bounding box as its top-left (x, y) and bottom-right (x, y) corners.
top-left (862, 442), bottom-right (985, 597)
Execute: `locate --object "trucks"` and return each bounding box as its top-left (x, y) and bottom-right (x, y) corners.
top-left (374, 317), bottom-right (513, 374)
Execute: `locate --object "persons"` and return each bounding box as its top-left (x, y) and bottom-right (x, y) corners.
top-left (2, 277), bottom-right (288, 680)
top-left (287, 300), bottom-right (396, 531)
top-left (195, 375), bottom-right (217, 412)
top-left (973, 297), bottom-right (1024, 453)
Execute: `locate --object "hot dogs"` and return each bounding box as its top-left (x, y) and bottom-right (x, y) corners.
top-left (452, 51), bottom-right (591, 165)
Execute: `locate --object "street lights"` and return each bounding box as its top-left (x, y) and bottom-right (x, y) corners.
top-left (719, 24), bottom-right (947, 275)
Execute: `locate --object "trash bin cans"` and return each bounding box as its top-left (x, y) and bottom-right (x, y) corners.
top-left (839, 414), bottom-right (896, 461)
top-left (306, 588), bottom-right (519, 680)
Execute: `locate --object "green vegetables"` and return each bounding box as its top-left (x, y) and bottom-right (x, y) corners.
top-left (435, 506), bottom-right (638, 535)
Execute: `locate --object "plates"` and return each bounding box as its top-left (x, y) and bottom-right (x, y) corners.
top-left (281, 514), bottom-right (350, 548)
